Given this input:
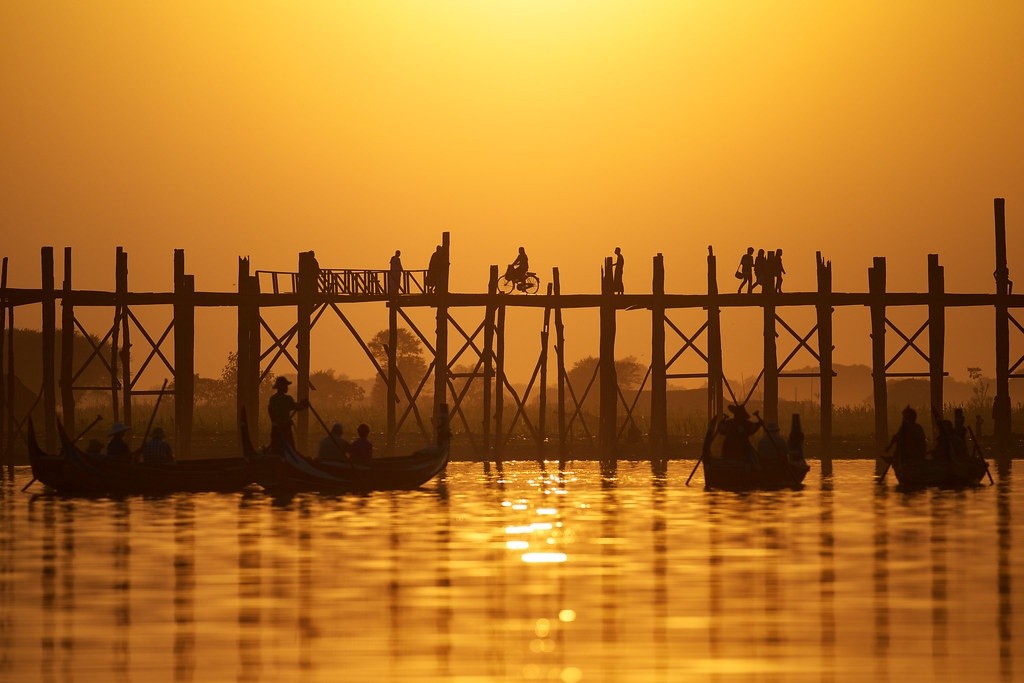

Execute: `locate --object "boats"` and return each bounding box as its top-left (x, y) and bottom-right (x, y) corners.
top-left (264, 432), bottom-right (452, 495)
top-left (701, 447), bottom-right (811, 492)
top-left (24, 411), bottom-right (266, 498)
top-left (882, 455), bottom-right (990, 494)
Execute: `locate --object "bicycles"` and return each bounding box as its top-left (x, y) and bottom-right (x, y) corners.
top-left (497, 263), bottom-right (541, 294)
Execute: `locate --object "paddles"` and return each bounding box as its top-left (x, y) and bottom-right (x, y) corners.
top-left (967, 425), bottom-right (993, 484)
top-left (753, 410), bottom-right (800, 486)
top-left (686, 413), bottom-right (729, 485)
top-left (264, 396), bottom-right (304, 455)
top-left (303, 397), bottom-right (365, 491)
top-left (22, 414), bottom-right (104, 491)
top-left (135, 378), bottom-right (168, 462)
top-left (878, 405), bottom-right (910, 485)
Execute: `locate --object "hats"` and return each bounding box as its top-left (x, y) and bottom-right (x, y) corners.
top-left (149, 427), bottom-right (166, 439)
top-left (86, 438), bottom-right (104, 452)
top-left (764, 421), bottom-right (780, 432)
top-left (937, 420), bottom-right (953, 435)
top-left (272, 376), bottom-right (292, 389)
top-left (728, 404), bottom-right (750, 420)
top-left (106, 422), bottom-right (132, 437)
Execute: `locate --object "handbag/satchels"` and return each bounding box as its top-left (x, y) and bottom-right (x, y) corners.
top-left (735, 271), bottom-right (744, 279)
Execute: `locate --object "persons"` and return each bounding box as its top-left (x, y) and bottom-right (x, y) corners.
top-left (511, 246), bottom-right (528, 292)
top-left (106, 422), bottom-right (134, 461)
top-left (736, 246), bottom-right (785, 293)
top-left (759, 422), bottom-right (789, 462)
top-left (612, 247), bottom-right (625, 295)
top-left (389, 249), bottom-right (404, 295)
top-left (425, 245), bottom-right (442, 295)
top-left (309, 250), bottom-right (322, 293)
top-left (993, 261), bottom-right (1013, 295)
top-left (267, 375), bottom-right (309, 457)
top-left (893, 404), bottom-right (968, 459)
top-left (319, 420), bottom-right (352, 463)
top-left (141, 429), bottom-right (175, 464)
top-left (87, 439), bottom-right (107, 464)
top-left (720, 402), bottom-right (762, 459)
top-left (351, 422), bottom-right (373, 462)
